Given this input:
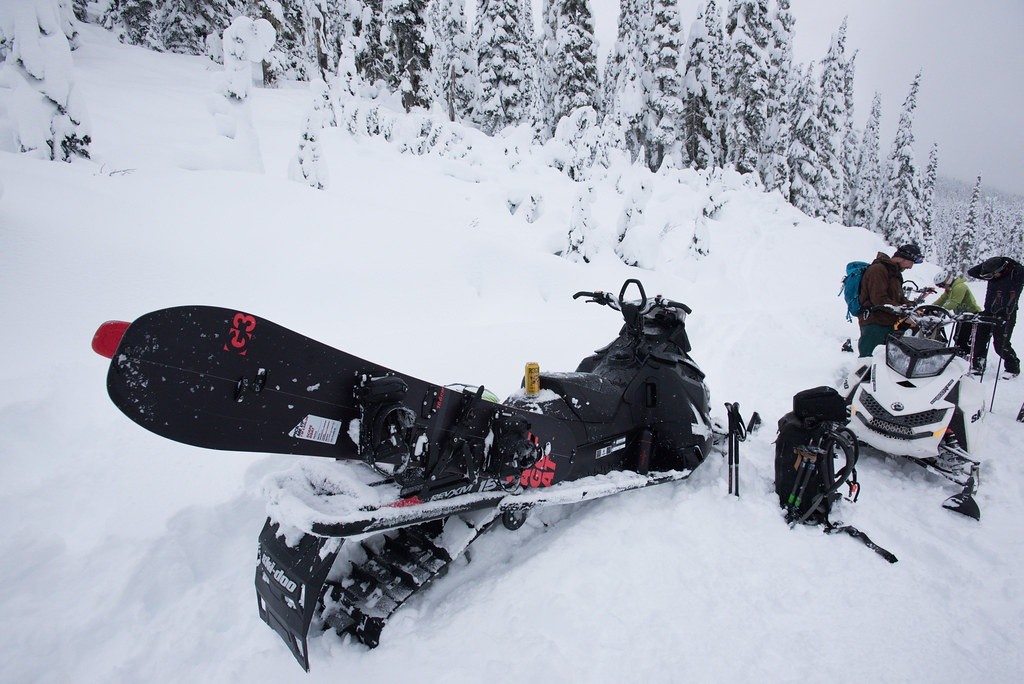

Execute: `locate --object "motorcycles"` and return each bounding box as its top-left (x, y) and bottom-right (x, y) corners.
top-left (823, 285), bottom-right (1011, 522)
top-left (90, 278), bottom-right (734, 669)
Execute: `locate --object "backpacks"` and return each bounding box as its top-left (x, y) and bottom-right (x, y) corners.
top-left (771, 386), bottom-right (861, 526)
top-left (838, 259), bottom-right (892, 323)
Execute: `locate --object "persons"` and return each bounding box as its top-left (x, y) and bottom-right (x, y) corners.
top-left (964, 256), bottom-right (1024, 381)
top-left (931, 270), bottom-right (982, 357)
top-left (838, 243), bottom-right (925, 358)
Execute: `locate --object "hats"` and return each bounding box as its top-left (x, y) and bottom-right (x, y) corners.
top-left (981, 257), bottom-right (1005, 275)
top-left (897, 244), bottom-right (923, 263)
top-left (934, 271), bottom-right (953, 284)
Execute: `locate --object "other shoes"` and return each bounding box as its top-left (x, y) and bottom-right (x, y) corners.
top-left (999, 370), bottom-right (1019, 380)
top-left (970, 369), bottom-right (986, 375)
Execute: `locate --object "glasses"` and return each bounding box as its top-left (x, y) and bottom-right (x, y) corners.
top-left (936, 271), bottom-right (952, 288)
top-left (979, 261), bottom-right (1008, 280)
top-left (903, 248), bottom-right (925, 263)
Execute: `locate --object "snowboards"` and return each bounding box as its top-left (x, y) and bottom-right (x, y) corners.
top-left (105, 304), bottom-right (578, 488)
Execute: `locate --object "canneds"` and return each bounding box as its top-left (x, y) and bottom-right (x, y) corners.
top-left (525, 362), bottom-right (539, 397)
